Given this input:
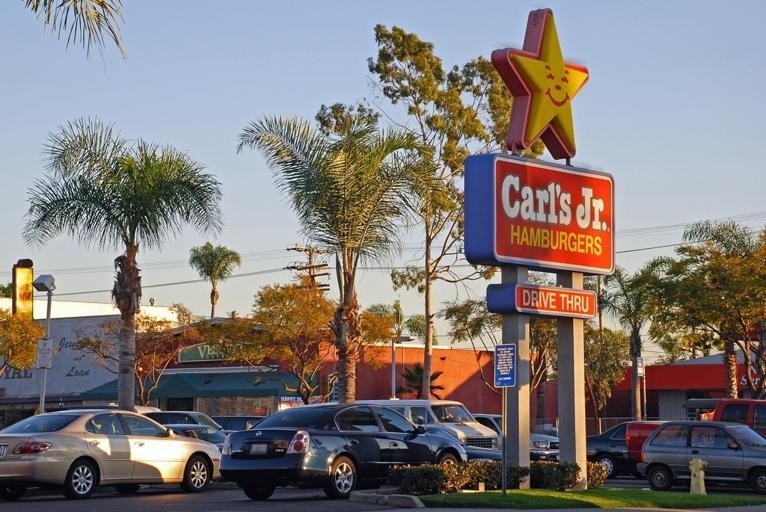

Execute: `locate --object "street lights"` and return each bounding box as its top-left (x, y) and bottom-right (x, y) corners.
top-left (389, 334), bottom-right (416, 400)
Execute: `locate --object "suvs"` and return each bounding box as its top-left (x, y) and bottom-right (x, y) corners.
top-left (330, 398), bottom-right (498, 451)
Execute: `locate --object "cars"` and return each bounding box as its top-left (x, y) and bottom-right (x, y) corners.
top-left (117, 411), bottom-right (268, 455)
top-left (458, 413), bottom-right (560, 461)
top-left (586, 399), bottom-right (766, 494)
top-left (0, 409), bottom-right (222, 500)
top-left (220, 403), bottom-right (468, 501)
